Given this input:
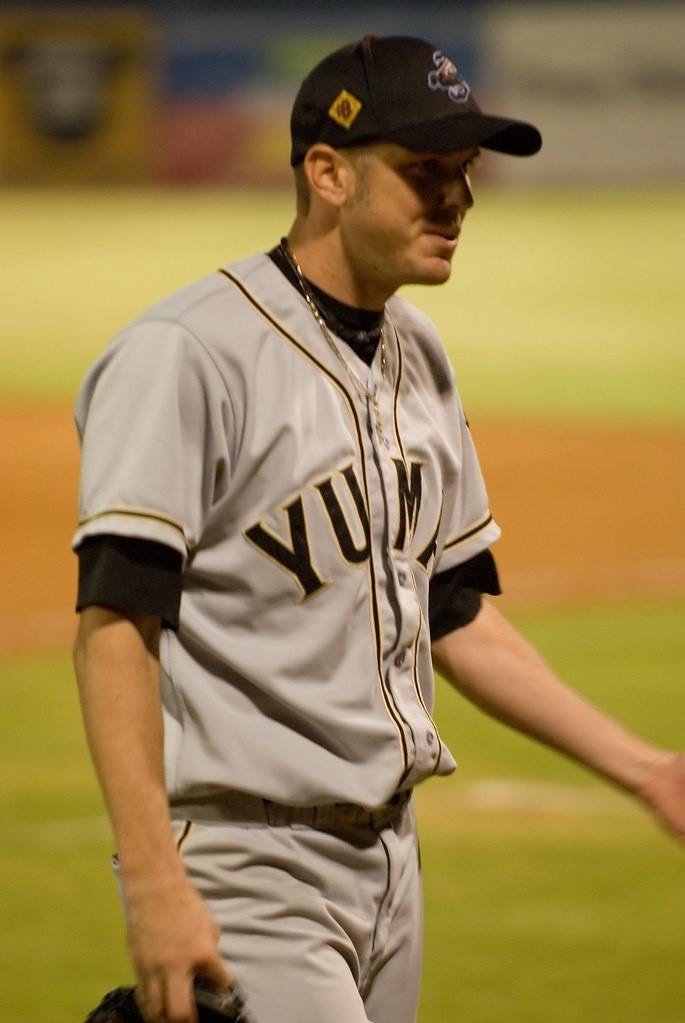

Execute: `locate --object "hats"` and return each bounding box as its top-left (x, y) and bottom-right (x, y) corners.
top-left (290, 34), bottom-right (544, 168)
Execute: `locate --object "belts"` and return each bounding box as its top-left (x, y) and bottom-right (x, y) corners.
top-left (267, 784), bottom-right (413, 831)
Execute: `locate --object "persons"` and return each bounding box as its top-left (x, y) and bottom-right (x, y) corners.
top-left (71, 34), bottom-right (685, 1023)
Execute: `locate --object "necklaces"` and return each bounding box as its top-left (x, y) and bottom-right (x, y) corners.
top-left (285, 244), bottom-right (386, 445)
